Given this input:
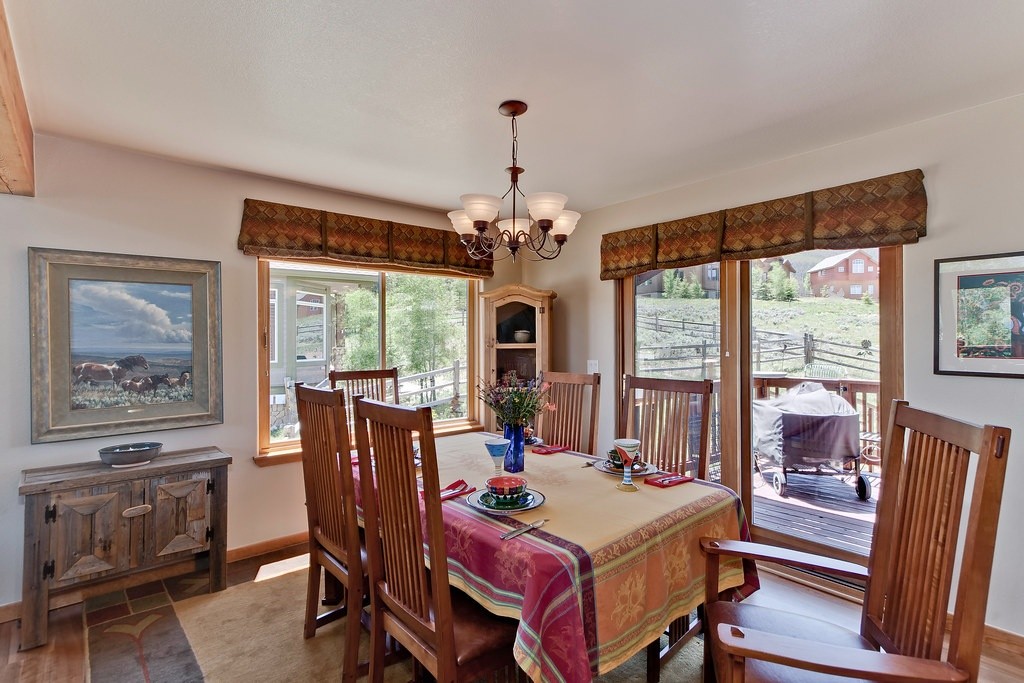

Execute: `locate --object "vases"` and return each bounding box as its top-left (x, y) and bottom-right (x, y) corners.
top-left (503, 423), bottom-right (524, 474)
top-left (515, 331), bottom-right (530, 343)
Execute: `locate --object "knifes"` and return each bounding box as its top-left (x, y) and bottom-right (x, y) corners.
top-left (504, 519), bottom-right (545, 541)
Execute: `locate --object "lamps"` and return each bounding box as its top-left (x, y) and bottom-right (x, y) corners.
top-left (448, 100), bottom-right (582, 265)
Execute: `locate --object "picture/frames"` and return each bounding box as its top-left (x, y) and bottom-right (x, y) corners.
top-left (28, 246), bottom-right (224, 446)
top-left (933, 251), bottom-right (1024, 378)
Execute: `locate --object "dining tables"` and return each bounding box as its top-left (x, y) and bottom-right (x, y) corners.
top-left (340, 431), bottom-right (761, 683)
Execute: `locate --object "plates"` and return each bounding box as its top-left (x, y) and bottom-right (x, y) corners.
top-left (593, 459), bottom-right (658, 476)
top-left (466, 486), bottom-right (545, 514)
top-left (524, 437), bottom-right (543, 447)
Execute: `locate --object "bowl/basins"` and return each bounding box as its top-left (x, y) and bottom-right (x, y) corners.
top-left (607, 448), bottom-right (641, 468)
top-left (484, 475), bottom-right (527, 503)
top-left (98, 442), bottom-right (163, 468)
top-left (524, 428), bottom-right (533, 440)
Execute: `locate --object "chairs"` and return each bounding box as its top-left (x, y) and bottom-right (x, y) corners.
top-left (295, 381), bottom-right (430, 683)
top-left (352, 393), bottom-right (534, 683)
top-left (329, 367), bottom-right (400, 449)
top-left (621, 374), bottom-right (716, 671)
top-left (542, 372), bottom-right (601, 455)
top-left (700, 398), bottom-right (1012, 683)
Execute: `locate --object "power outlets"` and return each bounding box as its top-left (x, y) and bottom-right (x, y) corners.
top-left (586, 360), bottom-right (598, 374)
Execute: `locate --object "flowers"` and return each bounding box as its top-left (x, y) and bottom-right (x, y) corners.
top-left (475, 369), bottom-right (557, 424)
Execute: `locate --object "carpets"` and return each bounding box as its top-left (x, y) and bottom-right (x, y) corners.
top-left (171, 565), bottom-right (706, 683)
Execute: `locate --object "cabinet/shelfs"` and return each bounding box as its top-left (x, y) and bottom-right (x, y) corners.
top-left (17, 445), bottom-right (233, 652)
top-left (479, 283), bottom-right (558, 438)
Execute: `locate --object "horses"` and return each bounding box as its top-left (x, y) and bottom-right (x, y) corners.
top-left (72, 353), bottom-right (150, 392)
top-left (128, 373), bottom-right (171, 398)
top-left (169, 371), bottom-right (190, 387)
top-left (122, 376), bottom-right (153, 393)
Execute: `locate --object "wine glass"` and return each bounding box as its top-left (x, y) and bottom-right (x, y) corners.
top-left (614, 438), bottom-right (640, 491)
top-left (483, 438), bottom-right (512, 478)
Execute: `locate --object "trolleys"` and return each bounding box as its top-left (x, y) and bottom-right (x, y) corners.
top-left (752, 382), bottom-right (872, 500)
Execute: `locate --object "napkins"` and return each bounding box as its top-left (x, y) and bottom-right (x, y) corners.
top-left (531, 445), bottom-right (571, 455)
top-left (643, 472), bottom-right (695, 489)
top-left (420, 479), bottom-right (477, 502)
